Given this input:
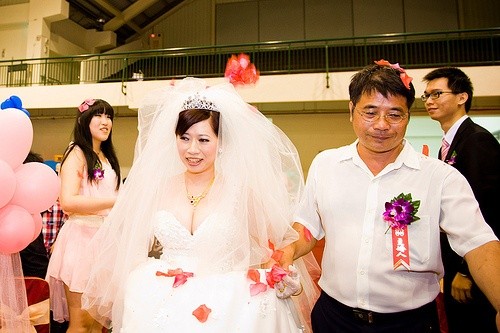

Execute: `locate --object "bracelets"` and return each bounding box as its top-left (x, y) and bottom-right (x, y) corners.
top-left (291, 283), bottom-right (303, 296)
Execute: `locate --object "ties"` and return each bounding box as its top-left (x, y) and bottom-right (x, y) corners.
top-left (441, 139), bottom-right (450, 161)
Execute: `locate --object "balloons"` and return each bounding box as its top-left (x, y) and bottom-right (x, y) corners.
top-left (0, 159), bottom-right (15, 209)
top-left (0, 108), bottom-right (33, 170)
top-left (9, 162), bottom-right (61, 215)
top-left (29, 212), bottom-right (42, 243)
top-left (0, 205), bottom-right (35, 255)
top-left (1, 95), bottom-right (31, 117)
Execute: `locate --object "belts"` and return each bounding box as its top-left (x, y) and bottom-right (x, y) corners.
top-left (321, 291), bottom-right (437, 328)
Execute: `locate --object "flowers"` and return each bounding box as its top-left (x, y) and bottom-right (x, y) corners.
top-left (446, 150), bottom-right (460, 165)
top-left (89, 165), bottom-right (107, 181)
top-left (381, 193), bottom-right (422, 234)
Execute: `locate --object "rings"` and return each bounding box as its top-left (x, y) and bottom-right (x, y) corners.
top-left (278, 286), bottom-right (285, 292)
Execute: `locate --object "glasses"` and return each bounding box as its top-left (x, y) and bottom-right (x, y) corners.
top-left (354, 105), bottom-right (410, 124)
top-left (421, 91), bottom-right (464, 102)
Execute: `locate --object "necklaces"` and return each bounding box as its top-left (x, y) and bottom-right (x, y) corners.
top-left (183, 170), bottom-right (215, 205)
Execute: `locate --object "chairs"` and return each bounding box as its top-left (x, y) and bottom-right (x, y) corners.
top-left (15, 276), bottom-right (53, 333)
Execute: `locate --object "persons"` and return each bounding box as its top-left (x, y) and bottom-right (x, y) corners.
top-left (421, 68), bottom-right (500, 333)
top-left (43, 198), bottom-right (68, 257)
top-left (47, 97), bottom-right (121, 333)
top-left (21, 153), bottom-right (71, 333)
top-left (267, 61), bottom-right (500, 333)
top-left (81, 76), bottom-right (322, 333)
